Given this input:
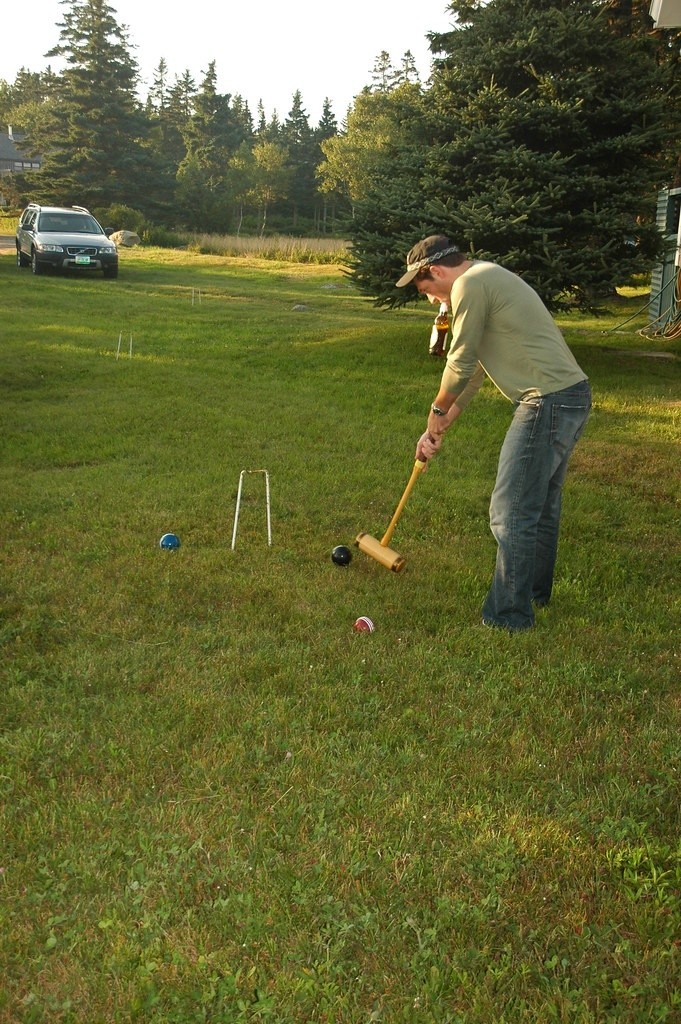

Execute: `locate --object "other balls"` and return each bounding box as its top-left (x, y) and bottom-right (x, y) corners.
top-left (160, 533), bottom-right (180, 549)
top-left (352, 614), bottom-right (376, 634)
top-left (331, 544), bottom-right (353, 568)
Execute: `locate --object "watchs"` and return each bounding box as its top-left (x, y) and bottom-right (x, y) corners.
top-left (430, 404), bottom-right (447, 416)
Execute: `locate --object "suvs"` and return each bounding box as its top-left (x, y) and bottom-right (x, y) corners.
top-left (15, 203), bottom-right (118, 279)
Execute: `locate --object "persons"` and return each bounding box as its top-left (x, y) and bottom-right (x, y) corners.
top-left (396, 235), bottom-right (592, 634)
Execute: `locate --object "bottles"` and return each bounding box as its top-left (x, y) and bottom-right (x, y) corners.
top-left (428, 303), bottom-right (450, 358)
